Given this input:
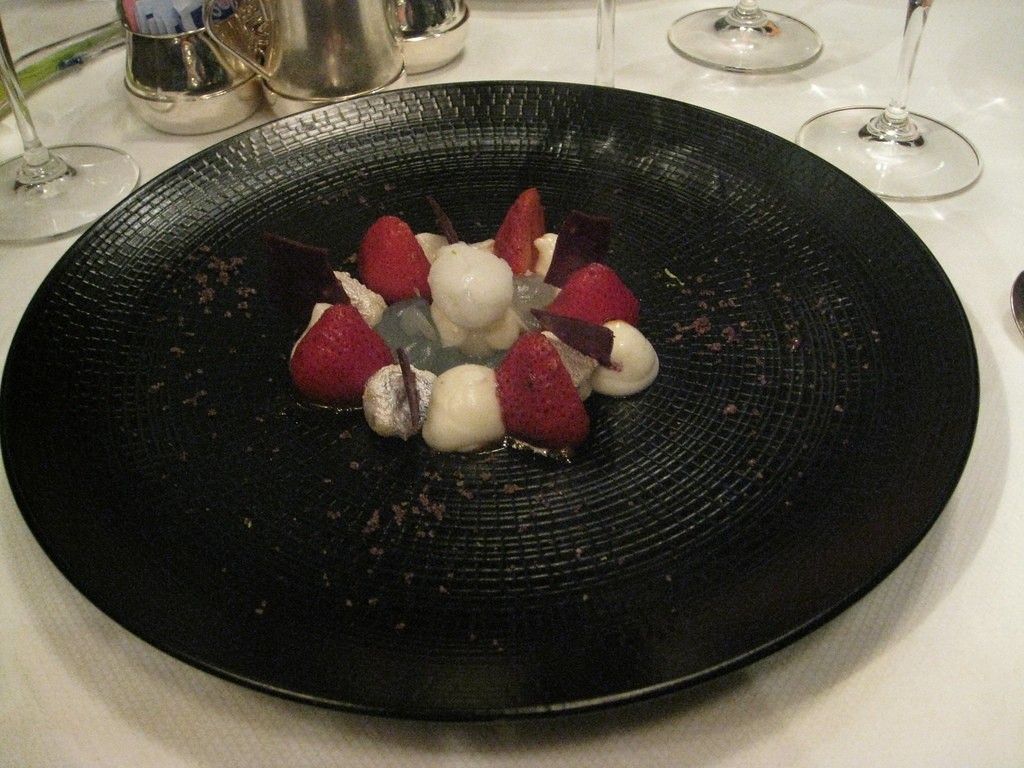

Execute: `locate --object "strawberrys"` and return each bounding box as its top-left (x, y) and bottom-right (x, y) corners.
top-left (286, 187), bottom-right (639, 448)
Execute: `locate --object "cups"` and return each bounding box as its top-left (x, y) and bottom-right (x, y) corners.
top-left (201, 0), bottom-right (407, 117)
top-left (123, 5), bottom-right (267, 136)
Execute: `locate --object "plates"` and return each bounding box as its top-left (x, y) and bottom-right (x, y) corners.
top-left (1, 79), bottom-right (980, 723)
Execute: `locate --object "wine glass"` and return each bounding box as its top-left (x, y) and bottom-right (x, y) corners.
top-left (794, 0), bottom-right (984, 201)
top-left (0, 22), bottom-right (142, 245)
top-left (667, 0), bottom-right (824, 76)
